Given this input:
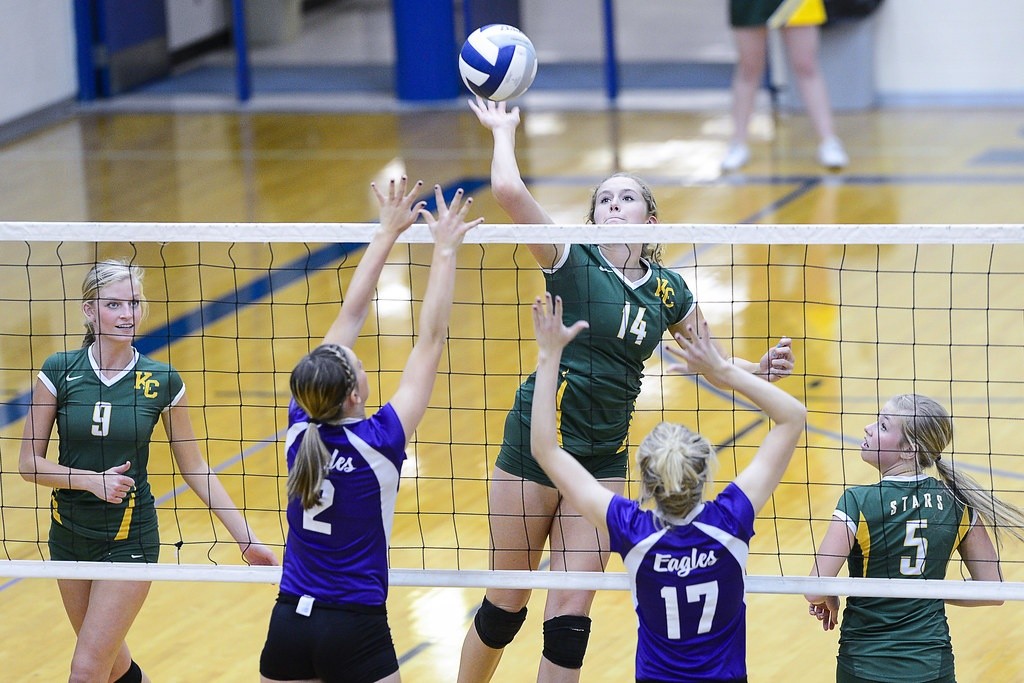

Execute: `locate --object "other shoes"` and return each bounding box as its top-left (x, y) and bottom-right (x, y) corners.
top-left (722, 141), bottom-right (749, 170)
top-left (818, 140), bottom-right (846, 167)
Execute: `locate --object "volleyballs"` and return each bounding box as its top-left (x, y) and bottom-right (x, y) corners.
top-left (457, 23), bottom-right (539, 103)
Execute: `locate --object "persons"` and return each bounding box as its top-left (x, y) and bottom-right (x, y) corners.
top-left (804, 394), bottom-right (1024, 683)
top-left (457, 94), bottom-right (807, 683)
top-left (719, 0), bottom-right (850, 173)
top-left (19, 258), bottom-right (279, 683)
top-left (260, 173), bottom-right (484, 683)
